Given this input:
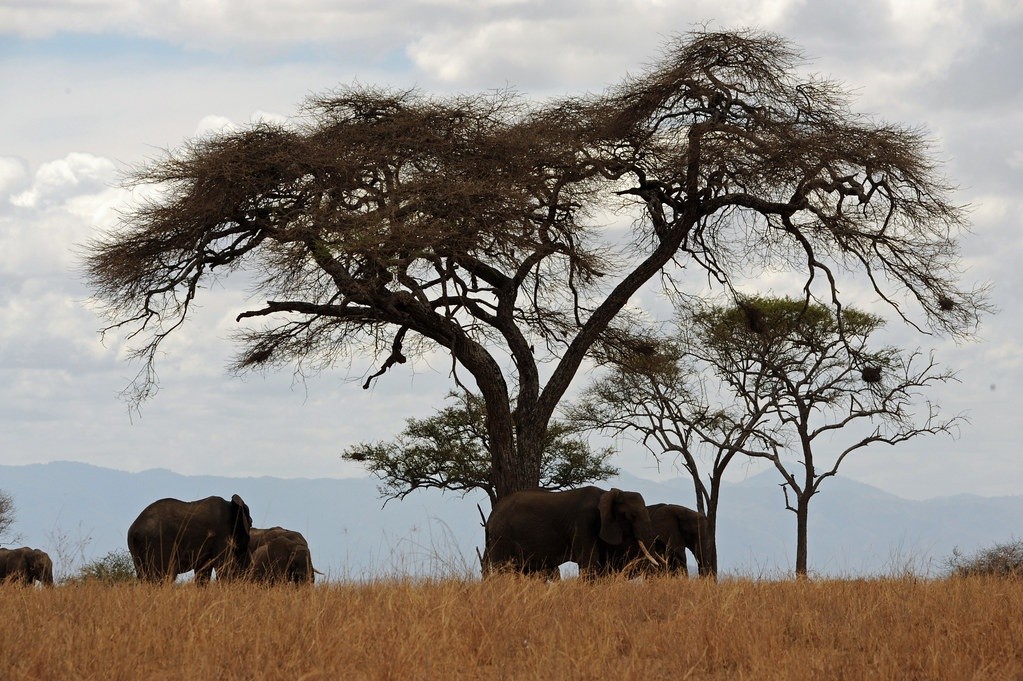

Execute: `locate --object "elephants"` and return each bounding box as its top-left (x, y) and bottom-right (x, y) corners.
top-left (486, 486), bottom-right (668, 582)
top-left (195, 526), bottom-right (326, 589)
top-left (0, 547), bottom-right (54, 587)
top-left (627, 503), bottom-right (711, 578)
top-left (127, 494), bottom-right (253, 586)
